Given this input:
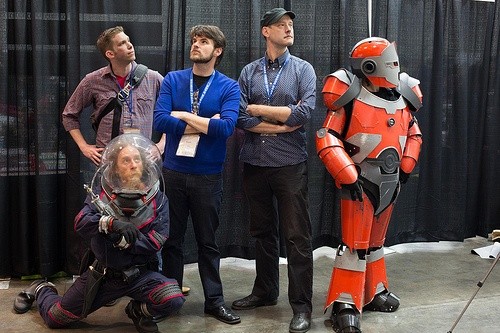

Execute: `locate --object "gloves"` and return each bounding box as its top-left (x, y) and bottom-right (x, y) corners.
top-left (341, 178), bottom-right (364, 202)
top-left (108, 217), bottom-right (143, 245)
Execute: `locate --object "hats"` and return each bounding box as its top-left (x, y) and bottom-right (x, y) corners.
top-left (260, 6), bottom-right (295, 27)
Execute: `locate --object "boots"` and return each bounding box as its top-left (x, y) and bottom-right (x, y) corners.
top-left (13, 278), bottom-right (58, 315)
top-left (125, 299), bottom-right (158, 333)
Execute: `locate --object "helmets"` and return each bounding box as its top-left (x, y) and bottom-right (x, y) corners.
top-left (349, 37), bottom-right (400, 88)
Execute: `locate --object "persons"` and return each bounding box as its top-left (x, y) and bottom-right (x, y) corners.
top-left (62, 26), bottom-right (192, 305)
top-left (232, 8), bottom-right (317, 333)
top-left (153, 25), bottom-right (240, 324)
top-left (13, 134), bottom-right (186, 333)
top-left (316, 36), bottom-right (423, 332)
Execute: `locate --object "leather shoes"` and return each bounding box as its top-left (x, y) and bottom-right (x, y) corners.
top-left (231, 293), bottom-right (278, 310)
top-left (288, 311), bottom-right (312, 333)
top-left (204, 304), bottom-right (241, 325)
